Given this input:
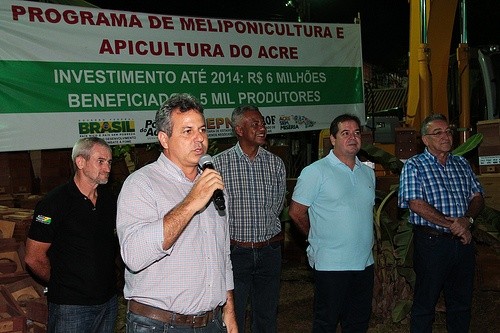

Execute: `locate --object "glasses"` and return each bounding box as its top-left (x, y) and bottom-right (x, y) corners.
top-left (425, 129), bottom-right (454, 137)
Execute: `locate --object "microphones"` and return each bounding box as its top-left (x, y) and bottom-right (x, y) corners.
top-left (198, 153), bottom-right (226, 211)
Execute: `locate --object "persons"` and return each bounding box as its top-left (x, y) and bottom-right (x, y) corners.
top-left (212, 105), bottom-right (287, 333)
top-left (24, 137), bottom-right (117, 333)
top-left (399, 114), bottom-right (485, 333)
top-left (289, 113), bottom-right (377, 333)
top-left (116, 93), bottom-right (238, 333)
top-left (357, 149), bottom-right (372, 162)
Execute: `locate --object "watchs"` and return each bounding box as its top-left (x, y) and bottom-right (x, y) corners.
top-left (464, 215), bottom-right (474, 226)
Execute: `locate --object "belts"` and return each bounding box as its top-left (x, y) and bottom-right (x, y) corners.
top-left (127, 299), bottom-right (222, 327)
top-left (414, 225), bottom-right (451, 238)
top-left (230, 231), bottom-right (284, 248)
